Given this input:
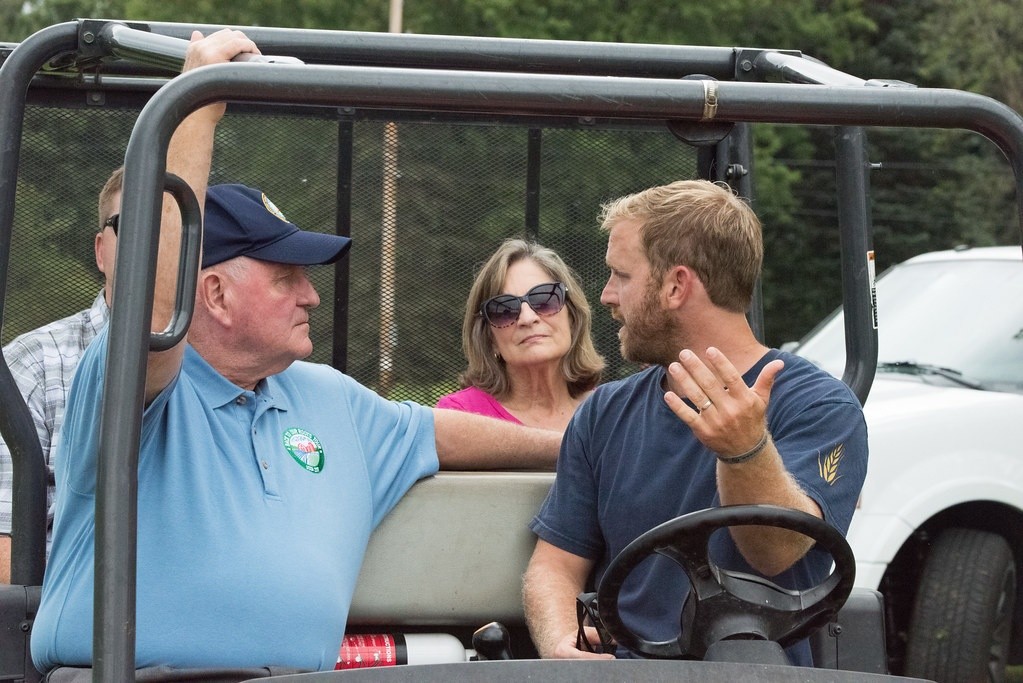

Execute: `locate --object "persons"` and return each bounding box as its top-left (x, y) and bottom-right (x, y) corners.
top-left (518, 178), bottom-right (870, 674)
top-left (28, 25), bottom-right (568, 683)
top-left (0, 164), bottom-right (126, 588)
top-left (433, 237), bottom-right (611, 472)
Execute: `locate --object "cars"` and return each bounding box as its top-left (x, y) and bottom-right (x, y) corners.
top-left (774, 242), bottom-right (1022, 680)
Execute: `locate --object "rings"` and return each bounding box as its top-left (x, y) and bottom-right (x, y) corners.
top-left (696, 400), bottom-right (712, 412)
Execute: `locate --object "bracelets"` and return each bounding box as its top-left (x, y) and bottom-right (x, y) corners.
top-left (714, 430), bottom-right (768, 464)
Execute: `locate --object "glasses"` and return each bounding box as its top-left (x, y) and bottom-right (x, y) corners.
top-left (477, 282), bottom-right (568, 328)
top-left (102, 213), bottom-right (119, 237)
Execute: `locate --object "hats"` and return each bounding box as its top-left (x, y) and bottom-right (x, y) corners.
top-left (201, 183), bottom-right (351, 271)
